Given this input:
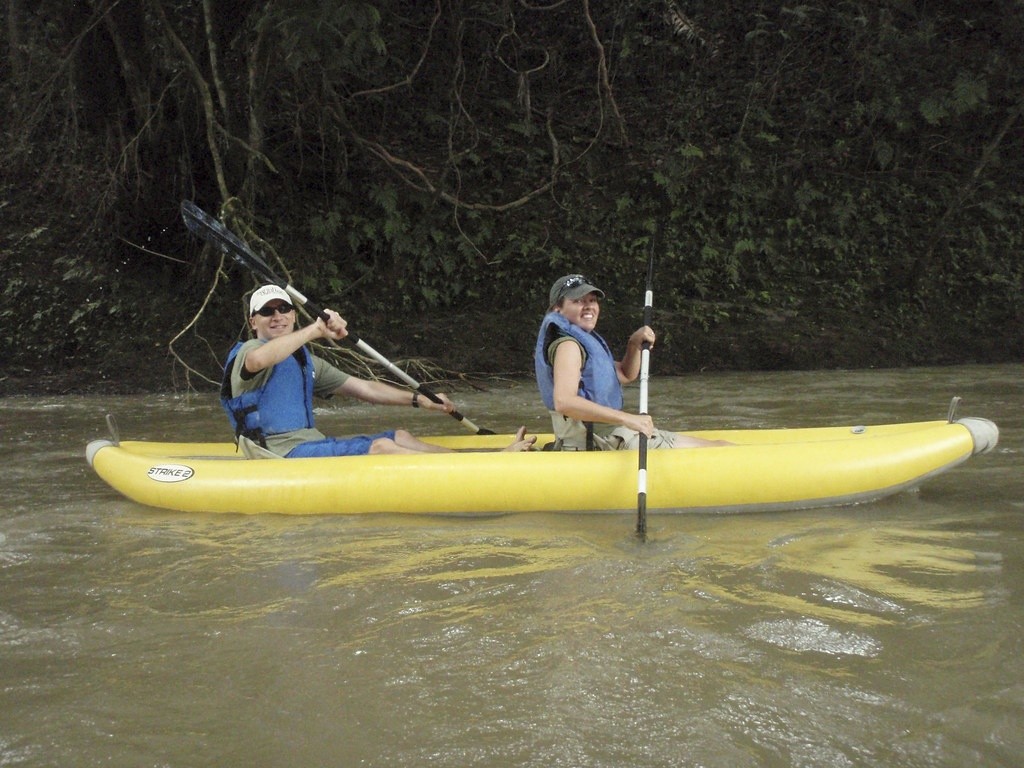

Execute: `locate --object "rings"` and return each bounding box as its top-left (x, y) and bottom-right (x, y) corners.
top-left (649, 332), bottom-right (654, 335)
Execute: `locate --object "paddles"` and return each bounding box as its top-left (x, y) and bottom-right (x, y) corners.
top-left (633, 237), bottom-right (656, 541)
top-left (178, 201), bottom-right (491, 434)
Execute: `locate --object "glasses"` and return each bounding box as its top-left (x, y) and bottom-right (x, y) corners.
top-left (251, 304), bottom-right (295, 317)
top-left (554, 277), bottom-right (594, 307)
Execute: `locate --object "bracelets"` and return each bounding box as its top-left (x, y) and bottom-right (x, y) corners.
top-left (412, 390), bottom-right (420, 408)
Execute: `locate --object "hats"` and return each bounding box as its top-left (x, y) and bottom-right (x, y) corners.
top-left (249, 284), bottom-right (293, 317)
top-left (549, 274), bottom-right (606, 308)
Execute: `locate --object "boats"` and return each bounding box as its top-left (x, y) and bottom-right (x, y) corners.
top-left (84, 397), bottom-right (1000, 519)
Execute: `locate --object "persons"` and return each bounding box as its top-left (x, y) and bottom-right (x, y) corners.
top-left (533, 275), bottom-right (737, 450)
top-left (230, 285), bottom-right (537, 459)
